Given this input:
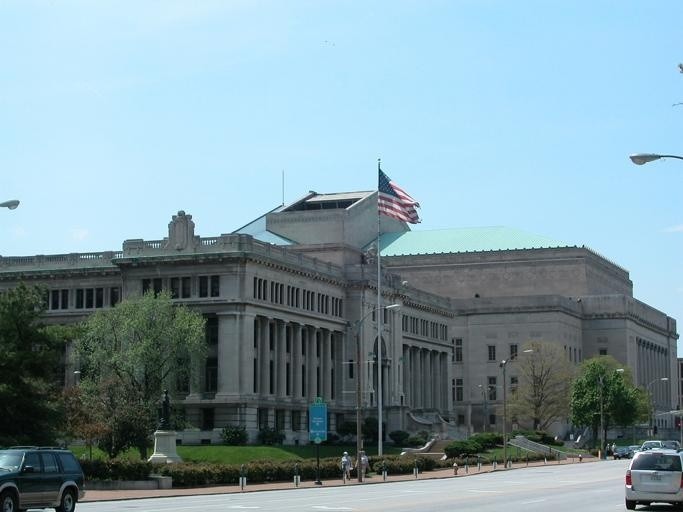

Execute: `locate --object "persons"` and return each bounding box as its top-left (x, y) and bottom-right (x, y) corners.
top-left (354, 449), bottom-right (371, 482)
top-left (611, 442), bottom-right (617, 455)
top-left (341, 452), bottom-right (353, 485)
top-left (606, 443), bottom-right (611, 456)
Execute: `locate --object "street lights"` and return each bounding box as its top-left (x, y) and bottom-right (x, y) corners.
top-left (504, 350), bottom-right (534, 468)
top-left (357, 303), bottom-right (401, 481)
top-left (598, 368), bottom-right (625, 459)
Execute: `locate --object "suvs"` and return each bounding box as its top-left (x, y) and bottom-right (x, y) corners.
top-left (624, 448), bottom-right (683, 512)
top-left (0, 445), bottom-right (87, 512)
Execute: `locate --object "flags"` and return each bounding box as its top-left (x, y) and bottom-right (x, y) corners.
top-left (377, 167), bottom-right (422, 225)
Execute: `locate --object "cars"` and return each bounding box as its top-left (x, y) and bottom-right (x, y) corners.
top-left (613, 446), bottom-right (634, 460)
top-left (639, 440), bottom-right (664, 452)
